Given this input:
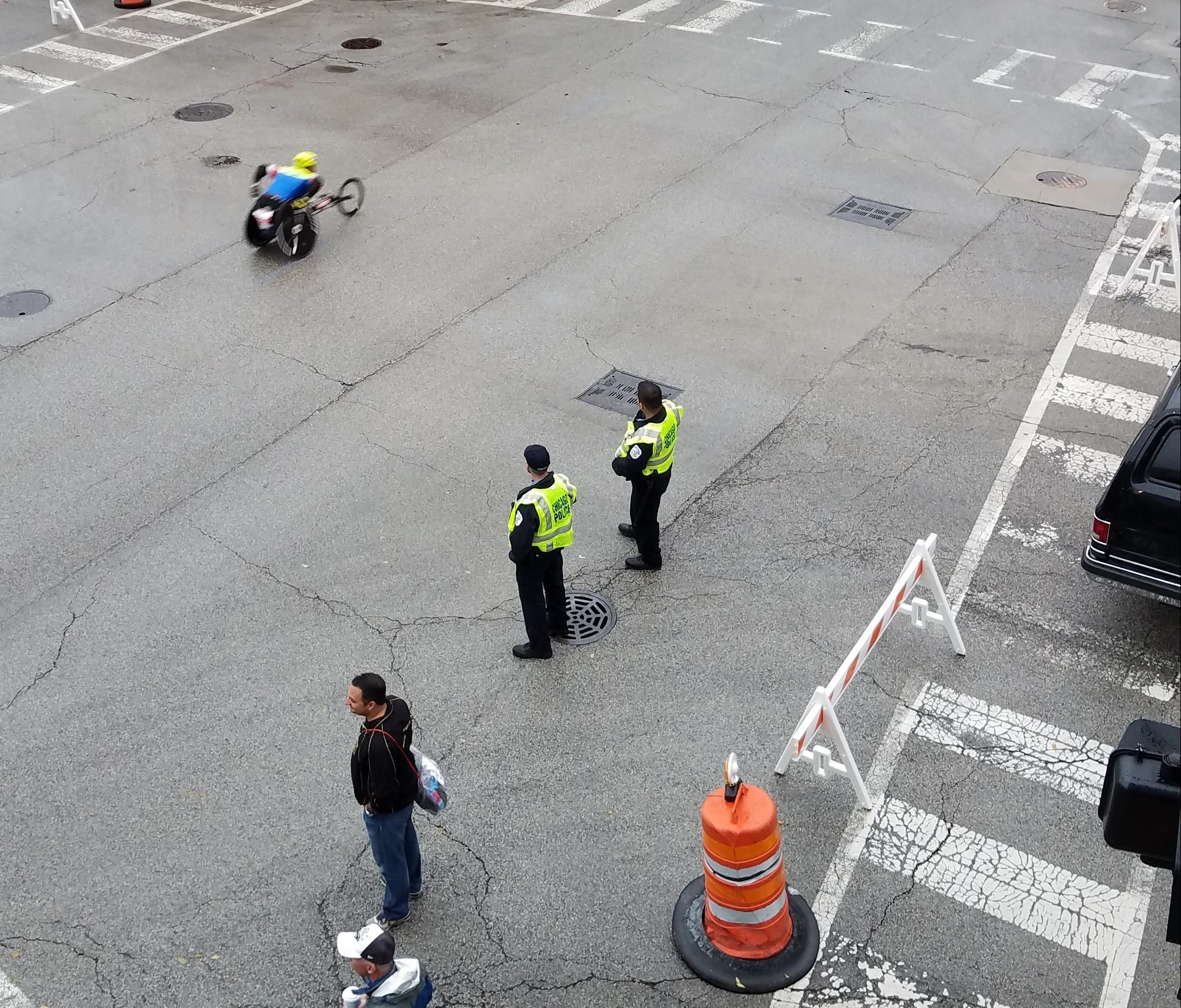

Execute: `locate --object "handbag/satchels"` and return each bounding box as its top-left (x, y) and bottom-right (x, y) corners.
top-left (409, 745), bottom-right (448, 816)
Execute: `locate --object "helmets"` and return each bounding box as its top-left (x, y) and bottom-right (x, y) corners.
top-left (292, 151), bottom-right (318, 167)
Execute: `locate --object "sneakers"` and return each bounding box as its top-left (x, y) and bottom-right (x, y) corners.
top-left (365, 908), bottom-right (412, 932)
top-left (379, 875), bottom-right (423, 899)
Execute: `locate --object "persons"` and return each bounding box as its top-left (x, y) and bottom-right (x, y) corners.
top-left (508, 444), bottom-right (577, 659)
top-left (345, 672), bottom-right (424, 932)
top-left (337, 923), bottom-right (433, 1008)
top-left (610, 382), bottom-right (676, 570)
top-left (249, 152), bottom-right (325, 237)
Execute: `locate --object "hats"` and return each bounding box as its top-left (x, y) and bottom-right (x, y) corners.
top-left (524, 444), bottom-right (550, 471)
top-left (337, 924), bottom-right (395, 965)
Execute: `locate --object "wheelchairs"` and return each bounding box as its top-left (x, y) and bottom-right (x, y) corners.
top-left (243, 172), bottom-right (366, 259)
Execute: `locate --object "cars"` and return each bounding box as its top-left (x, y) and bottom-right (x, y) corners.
top-left (1080, 360), bottom-right (1181, 607)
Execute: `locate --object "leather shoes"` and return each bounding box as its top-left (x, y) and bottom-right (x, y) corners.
top-left (618, 523), bottom-right (635, 538)
top-left (512, 642), bottom-right (553, 659)
top-left (546, 614), bottom-right (568, 635)
top-left (625, 555), bottom-right (662, 570)
